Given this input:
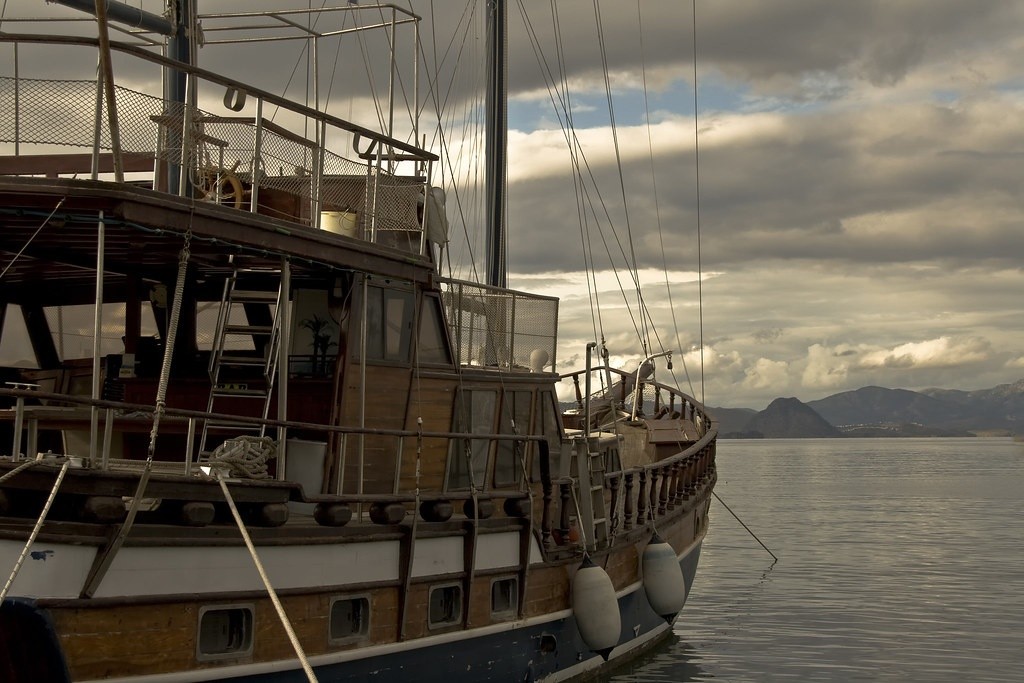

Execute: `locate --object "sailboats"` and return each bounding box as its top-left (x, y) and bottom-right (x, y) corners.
top-left (0, 0), bottom-right (718, 683)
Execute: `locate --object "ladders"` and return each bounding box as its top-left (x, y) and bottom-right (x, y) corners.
top-left (553, 436), bottom-right (610, 552)
